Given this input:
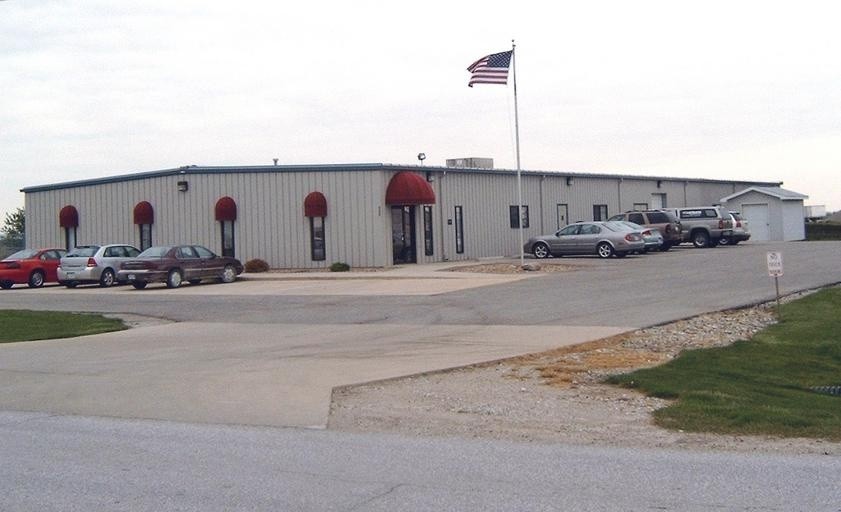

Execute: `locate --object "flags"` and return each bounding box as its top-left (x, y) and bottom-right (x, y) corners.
top-left (467, 50), bottom-right (512, 87)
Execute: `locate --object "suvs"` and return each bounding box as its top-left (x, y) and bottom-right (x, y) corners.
top-left (719, 210), bottom-right (751, 245)
top-left (647, 206), bottom-right (734, 248)
top-left (608, 209), bottom-right (683, 251)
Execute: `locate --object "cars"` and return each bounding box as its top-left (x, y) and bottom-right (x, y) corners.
top-left (392, 226), bottom-right (463, 263)
top-left (0, 247), bottom-right (71, 289)
top-left (117, 244), bottom-right (245, 289)
top-left (57, 243), bottom-right (143, 288)
top-left (524, 222), bottom-right (646, 258)
top-left (311, 228), bottom-right (324, 259)
top-left (592, 221), bottom-right (665, 254)
